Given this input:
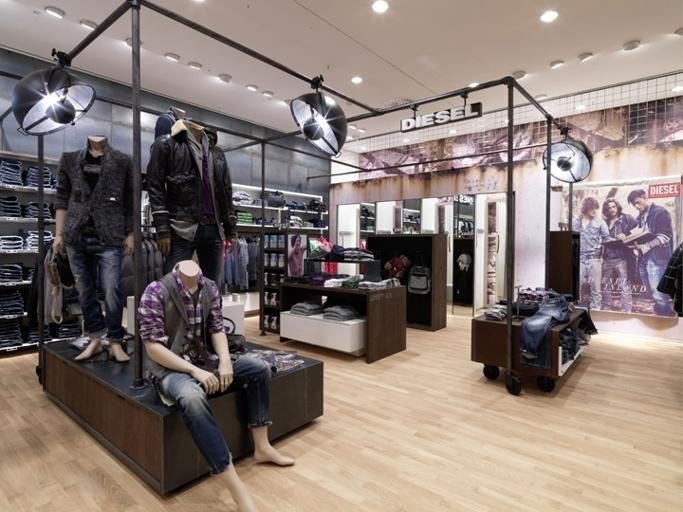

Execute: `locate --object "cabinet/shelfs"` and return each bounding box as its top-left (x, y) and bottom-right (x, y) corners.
top-left (278, 281), bottom-right (407, 363)
top-left (360, 233), bottom-right (447, 332)
top-left (472, 301), bottom-right (591, 395)
top-left (303, 258), bottom-right (382, 281)
top-left (0, 150), bottom-right (125, 351)
top-left (260, 230), bottom-right (310, 336)
top-left (550, 231), bottom-right (581, 304)
top-left (140, 170), bottom-right (330, 314)
top-left (42, 329), bottom-right (324, 497)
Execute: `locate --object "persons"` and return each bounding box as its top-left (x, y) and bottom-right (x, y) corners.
top-left (137, 259), bottom-right (295, 512)
top-left (288, 234), bottom-right (306, 277)
top-left (154, 106), bottom-right (186, 139)
top-left (145, 118), bottom-right (238, 280)
top-left (52, 134), bottom-right (133, 363)
top-left (600, 198), bottom-right (638, 313)
top-left (627, 188), bottom-right (673, 316)
top-left (570, 195), bottom-right (610, 309)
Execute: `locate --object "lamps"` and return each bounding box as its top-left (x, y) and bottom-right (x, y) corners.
top-left (12, 66), bottom-right (97, 137)
top-left (290, 94), bottom-right (347, 157)
top-left (542, 137), bottom-right (592, 183)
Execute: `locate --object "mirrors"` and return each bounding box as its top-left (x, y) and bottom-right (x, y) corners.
top-left (360, 195), bottom-right (475, 241)
